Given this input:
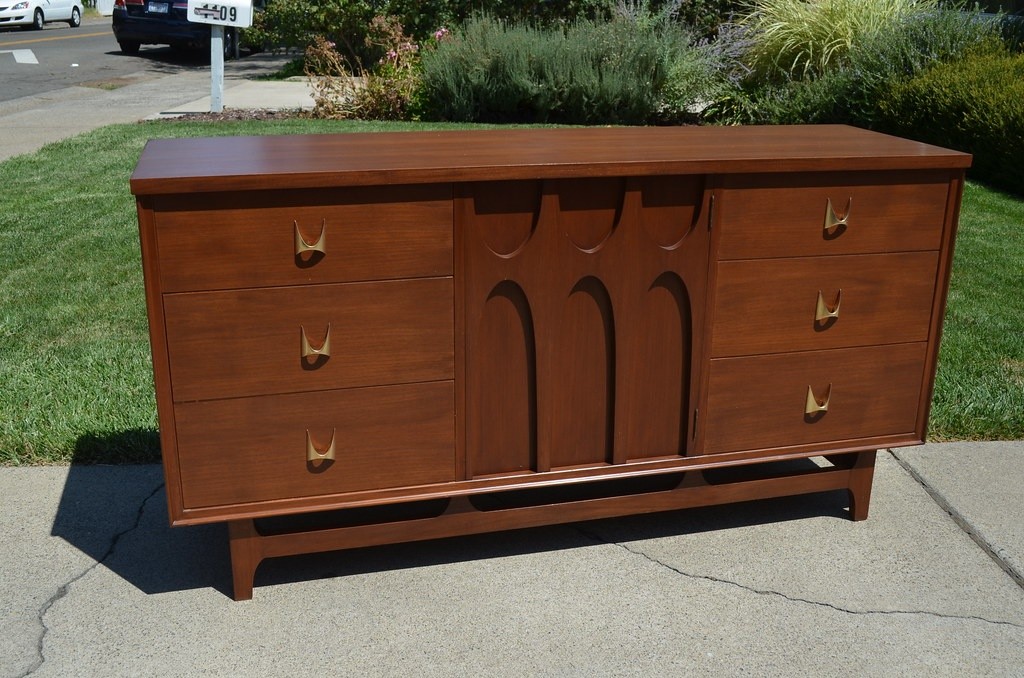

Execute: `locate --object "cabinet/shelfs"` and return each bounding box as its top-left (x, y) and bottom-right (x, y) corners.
top-left (130, 124), bottom-right (973, 602)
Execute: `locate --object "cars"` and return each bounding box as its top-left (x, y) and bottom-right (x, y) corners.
top-left (0, 0), bottom-right (85, 30)
top-left (113, 0), bottom-right (233, 60)
